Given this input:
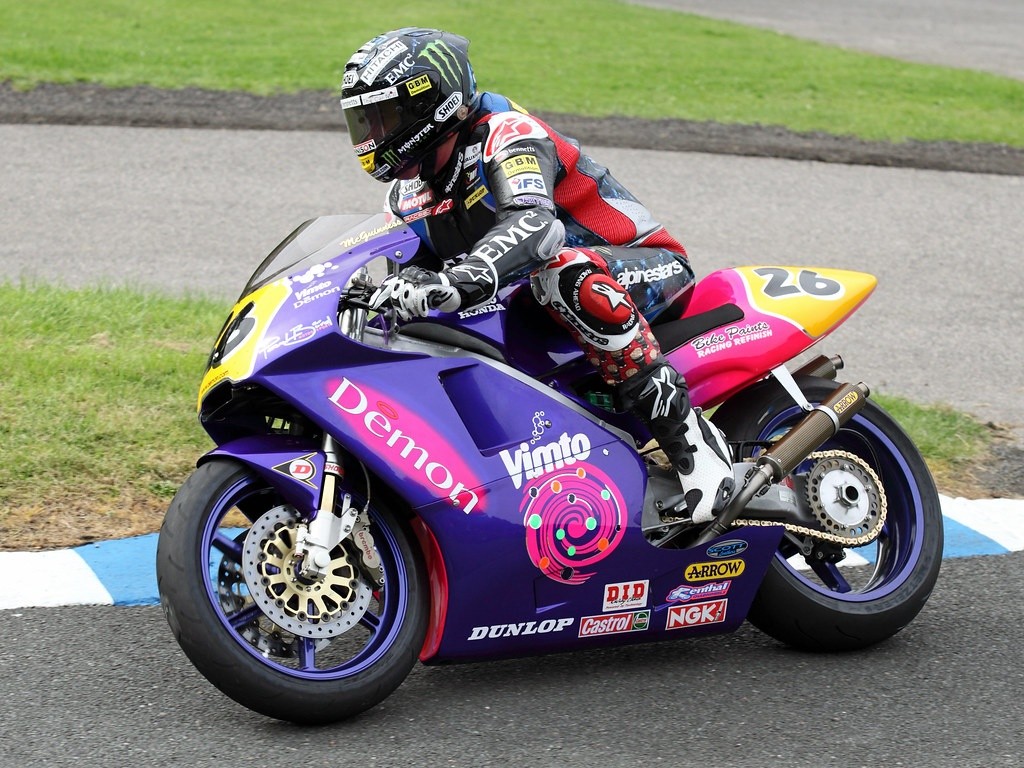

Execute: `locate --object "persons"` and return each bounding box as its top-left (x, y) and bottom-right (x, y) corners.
top-left (338, 26), bottom-right (733, 524)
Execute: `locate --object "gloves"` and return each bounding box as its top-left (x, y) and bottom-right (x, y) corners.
top-left (370, 265), bottom-right (464, 325)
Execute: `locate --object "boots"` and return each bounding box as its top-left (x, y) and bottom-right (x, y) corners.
top-left (618, 355), bottom-right (737, 528)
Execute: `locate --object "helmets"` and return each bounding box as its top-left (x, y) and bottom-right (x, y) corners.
top-left (339, 26), bottom-right (482, 183)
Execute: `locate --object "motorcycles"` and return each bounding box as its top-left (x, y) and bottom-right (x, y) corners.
top-left (155, 211), bottom-right (946, 726)
